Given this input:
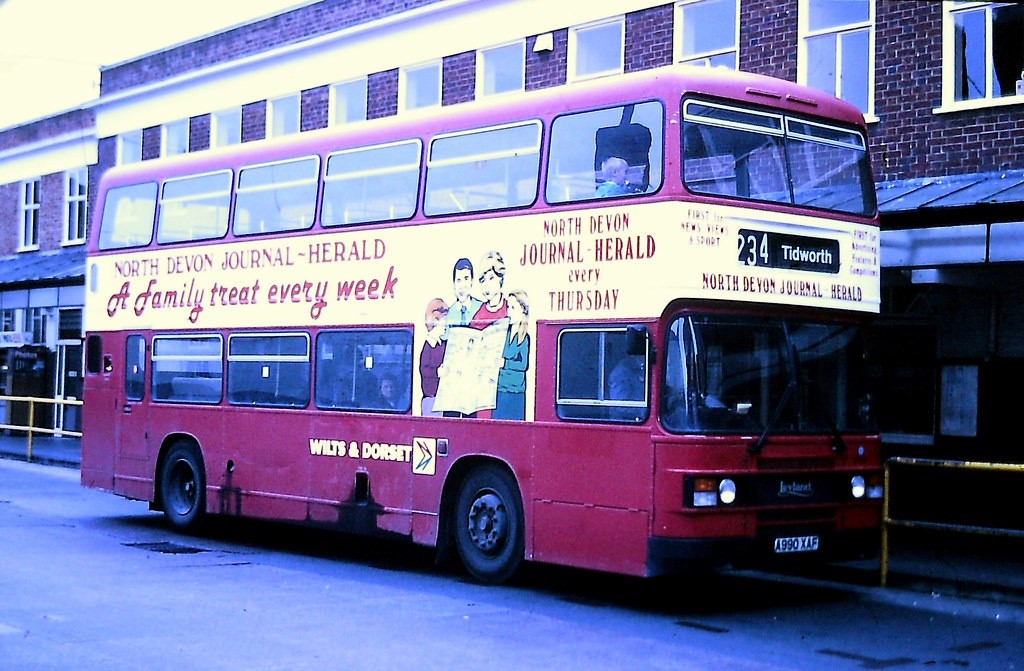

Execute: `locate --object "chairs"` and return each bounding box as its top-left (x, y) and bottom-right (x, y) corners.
top-left (106, 174), bottom-right (583, 238)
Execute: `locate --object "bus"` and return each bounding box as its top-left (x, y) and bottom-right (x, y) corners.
top-left (78, 64), bottom-right (888, 585)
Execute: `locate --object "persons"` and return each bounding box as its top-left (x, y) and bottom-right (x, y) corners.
top-left (596, 155), bottom-right (635, 197)
top-left (609, 352), bottom-right (655, 423)
